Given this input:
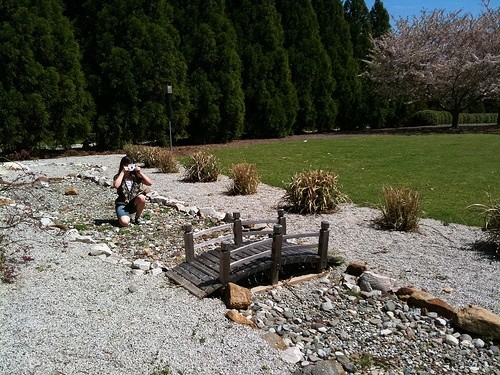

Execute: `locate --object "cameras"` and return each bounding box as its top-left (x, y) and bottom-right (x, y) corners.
top-left (127, 164), bottom-right (136, 171)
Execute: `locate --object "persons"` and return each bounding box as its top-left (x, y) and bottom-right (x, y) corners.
top-left (113, 155), bottom-right (153, 226)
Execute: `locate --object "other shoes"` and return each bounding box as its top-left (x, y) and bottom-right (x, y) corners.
top-left (135, 215), bottom-right (142, 224)
